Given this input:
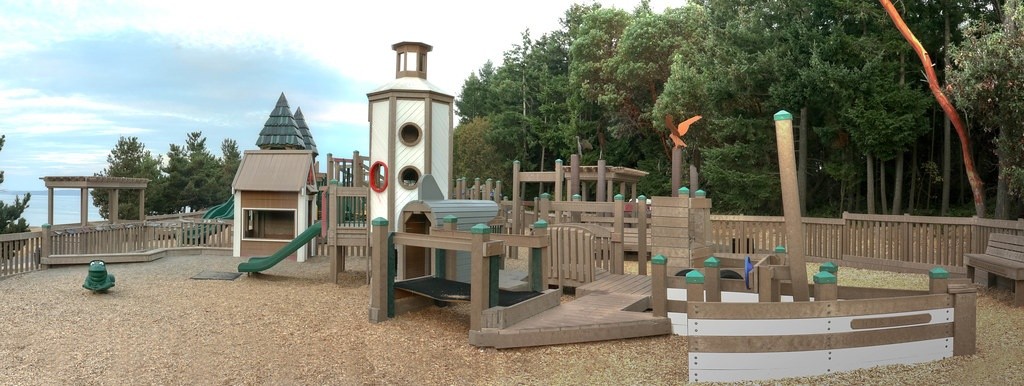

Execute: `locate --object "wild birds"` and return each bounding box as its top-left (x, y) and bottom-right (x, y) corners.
top-left (665, 113), bottom-right (703, 149)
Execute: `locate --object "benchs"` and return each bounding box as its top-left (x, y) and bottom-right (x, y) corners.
top-left (964, 232), bottom-right (1024, 308)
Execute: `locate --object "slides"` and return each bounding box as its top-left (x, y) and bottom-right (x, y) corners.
top-left (237, 222), bottom-right (322, 273)
top-left (182, 194), bottom-right (234, 244)
